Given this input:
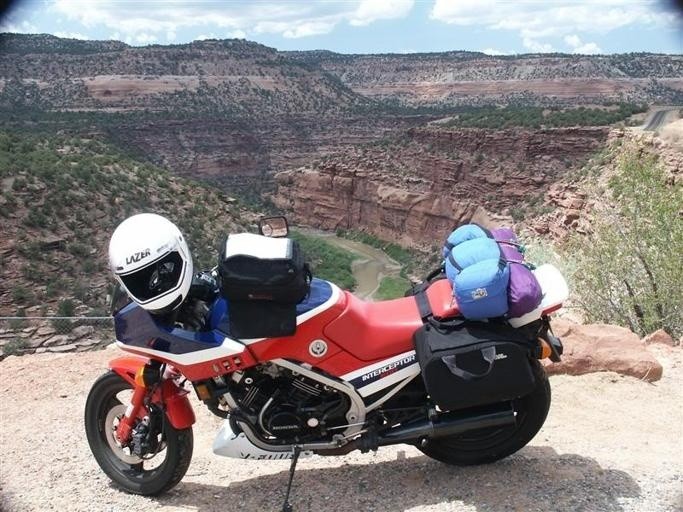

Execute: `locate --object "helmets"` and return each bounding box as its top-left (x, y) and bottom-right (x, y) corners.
top-left (107, 213), bottom-right (194, 317)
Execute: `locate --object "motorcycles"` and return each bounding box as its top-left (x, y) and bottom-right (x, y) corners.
top-left (83, 212), bottom-right (570, 512)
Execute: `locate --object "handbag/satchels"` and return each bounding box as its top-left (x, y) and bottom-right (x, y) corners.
top-left (413, 318), bottom-right (536, 411)
top-left (217, 232), bottom-right (313, 306)
